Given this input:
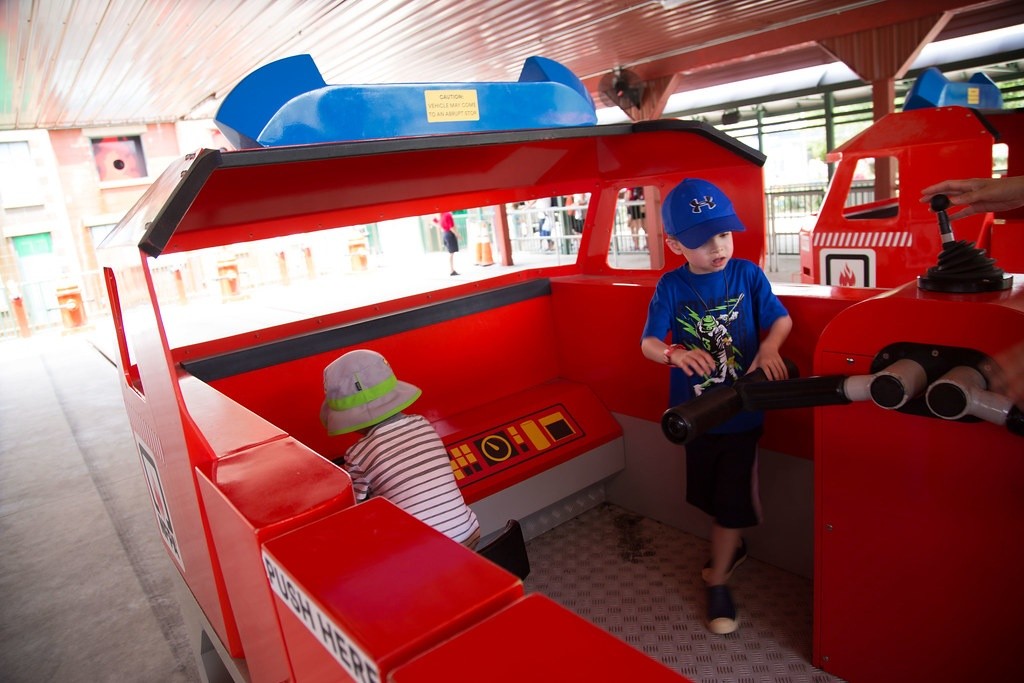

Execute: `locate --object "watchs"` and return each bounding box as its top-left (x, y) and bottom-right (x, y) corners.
top-left (663, 343), bottom-right (685, 369)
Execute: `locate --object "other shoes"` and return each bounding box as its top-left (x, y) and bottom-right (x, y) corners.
top-left (701, 535), bottom-right (750, 585)
top-left (450, 270), bottom-right (460, 276)
top-left (706, 589), bottom-right (739, 635)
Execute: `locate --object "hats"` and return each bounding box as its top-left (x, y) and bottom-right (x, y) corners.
top-left (319, 349), bottom-right (423, 436)
top-left (660, 178), bottom-right (745, 249)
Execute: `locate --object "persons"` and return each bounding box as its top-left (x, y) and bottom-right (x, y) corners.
top-left (642, 178), bottom-right (792, 635)
top-left (321, 349), bottom-right (481, 552)
top-left (432, 179), bottom-right (649, 277)
